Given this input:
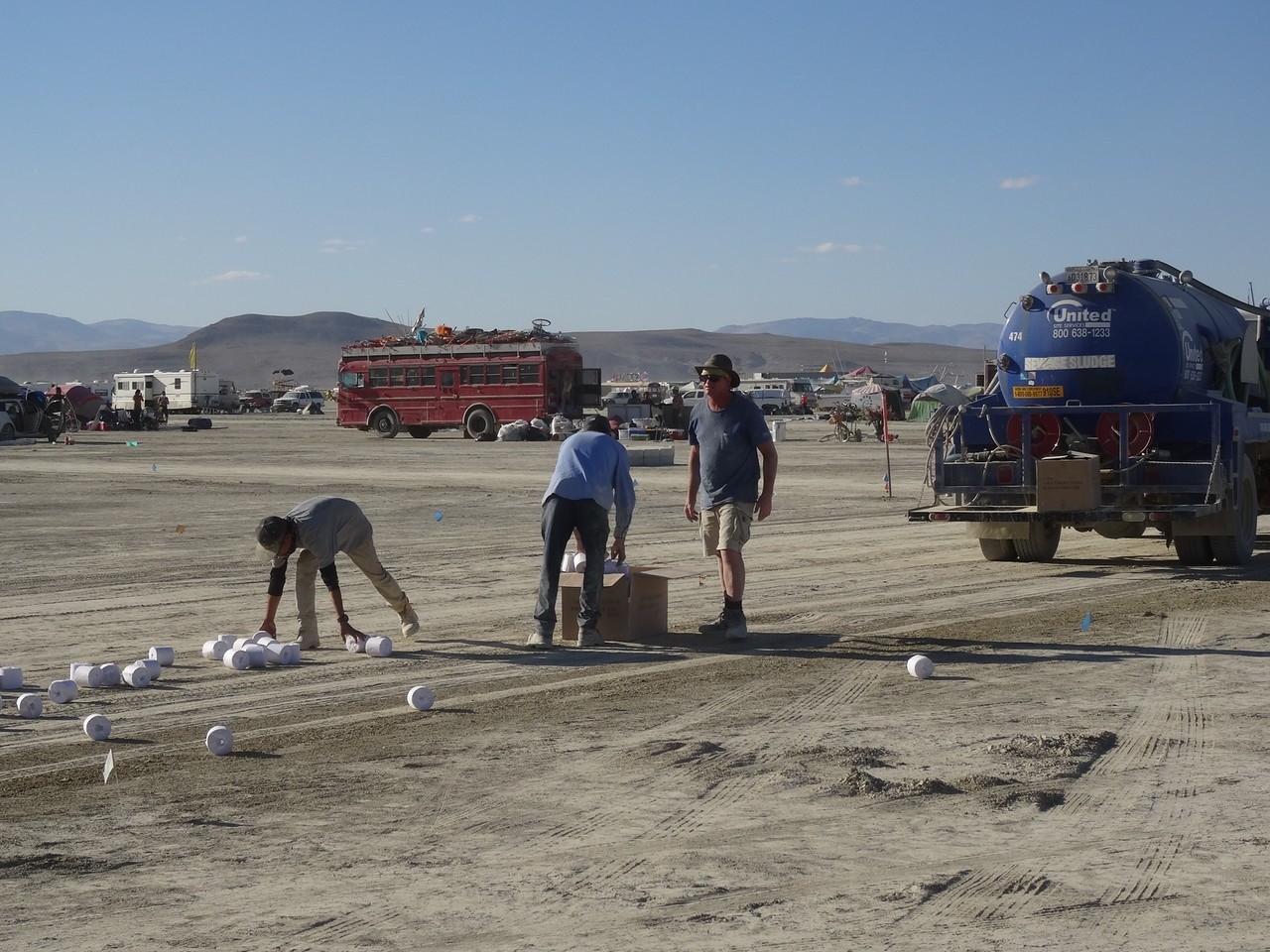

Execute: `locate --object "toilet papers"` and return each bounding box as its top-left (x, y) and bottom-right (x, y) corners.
top-left (100, 663), bottom-right (120, 686)
top-left (407, 686), bottom-right (435, 711)
top-left (561, 552), bottom-right (586, 573)
top-left (218, 635), bottom-right (237, 648)
top-left (83, 714), bottom-right (112, 740)
top-left (365, 636), bottom-right (392, 657)
top-left (202, 641), bottom-right (228, 660)
top-left (907, 654), bottom-right (935, 679)
top-left (70, 663), bottom-right (105, 687)
top-left (346, 633), bottom-right (370, 653)
top-left (223, 649), bottom-right (249, 669)
top-left (48, 680), bottom-right (78, 704)
top-left (149, 647), bottom-right (174, 666)
top-left (135, 660), bottom-right (162, 680)
top-left (206, 725), bottom-right (233, 755)
top-left (233, 630), bottom-right (301, 667)
top-left (122, 666), bottom-right (151, 687)
top-left (0, 667), bottom-right (23, 689)
top-left (16, 693), bottom-right (43, 719)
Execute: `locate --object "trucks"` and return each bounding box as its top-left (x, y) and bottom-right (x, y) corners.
top-left (219, 381), bottom-right (240, 411)
top-left (773, 377), bottom-right (816, 408)
top-left (17, 380), bottom-right (114, 405)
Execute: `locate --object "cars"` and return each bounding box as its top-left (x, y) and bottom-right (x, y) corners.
top-left (814, 406), bottom-right (836, 418)
top-left (0, 399), bottom-right (66, 440)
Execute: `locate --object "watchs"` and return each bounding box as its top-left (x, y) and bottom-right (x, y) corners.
top-left (337, 614), bottom-right (348, 622)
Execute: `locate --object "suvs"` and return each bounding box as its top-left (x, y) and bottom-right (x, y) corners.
top-left (272, 390), bottom-right (325, 414)
top-left (602, 392), bottom-right (631, 405)
top-left (241, 389), bottom-right (273, 408)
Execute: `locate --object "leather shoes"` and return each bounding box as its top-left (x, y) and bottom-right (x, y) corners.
top-left (290, 629), bottom-right (320, 650)
top-left (397, 602), bottom-right (420, 639)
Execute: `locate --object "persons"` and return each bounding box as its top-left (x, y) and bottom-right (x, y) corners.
top-left (47, 386), bottom-right (68, 446)
top-left (627, 385), bottom-right (684, 428)
top-left (133, 389), bottom-right (144, 410)
top-left (685, 354), bottom-right (777, 637)
top-left (524, 414), bottom-right (637, 649)
top-left (254, 496), bottom-right (420, 650)
top-left (156, 391), bottom-right (169, 424)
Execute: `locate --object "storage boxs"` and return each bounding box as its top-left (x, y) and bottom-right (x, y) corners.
top-left (626, 446), bottom-right (675, 466)
top-left (1034, 450), bottom-right (1101, 512)
top-left (531, 566), bottom-right (695, 642)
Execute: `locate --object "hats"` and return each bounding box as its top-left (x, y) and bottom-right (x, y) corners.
top-left (254, 515), bottom-right (288, 561)
top-left (694, 354), bottom-right (740, 389)
top-left (673, 387), bottom-right (678, 390)
top-left (585, 415), bottom-right (613, 437)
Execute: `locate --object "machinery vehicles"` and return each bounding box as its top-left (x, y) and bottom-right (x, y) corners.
top-left (906, 261), bottom-right (1270, 566)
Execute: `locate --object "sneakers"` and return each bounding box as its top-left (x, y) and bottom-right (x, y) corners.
top-left (728, 608), bottom-right (748, 639)
top-left (698, 607), bottom-right (728, 633)
top-left (525, 631), bottom-right (553, 648)
top-left (578, 629), bottom-right (606, 647)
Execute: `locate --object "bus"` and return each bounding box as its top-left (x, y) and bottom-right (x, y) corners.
top-left (332, 349), bottom-right (602, 439)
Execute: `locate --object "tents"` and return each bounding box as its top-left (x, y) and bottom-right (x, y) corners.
top-left (838, 365), bottom-right (983, 423)
top-left (45, 385), bottom-right (106, 430)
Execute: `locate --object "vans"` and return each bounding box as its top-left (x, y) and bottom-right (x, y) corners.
top-left (661, 389), bottom-right (704, 407)
top-left (744, 389), bottom-right (791, 414)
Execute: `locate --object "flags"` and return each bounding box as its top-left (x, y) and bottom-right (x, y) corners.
top-left (189, 342), bottom-right (195, 368)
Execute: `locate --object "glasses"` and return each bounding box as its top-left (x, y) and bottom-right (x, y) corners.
top-left (699, 374), bottom-right (729, 381)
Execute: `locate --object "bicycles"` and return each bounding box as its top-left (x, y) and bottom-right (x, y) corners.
top-left (818, 401), bottom-right (868, 444)
